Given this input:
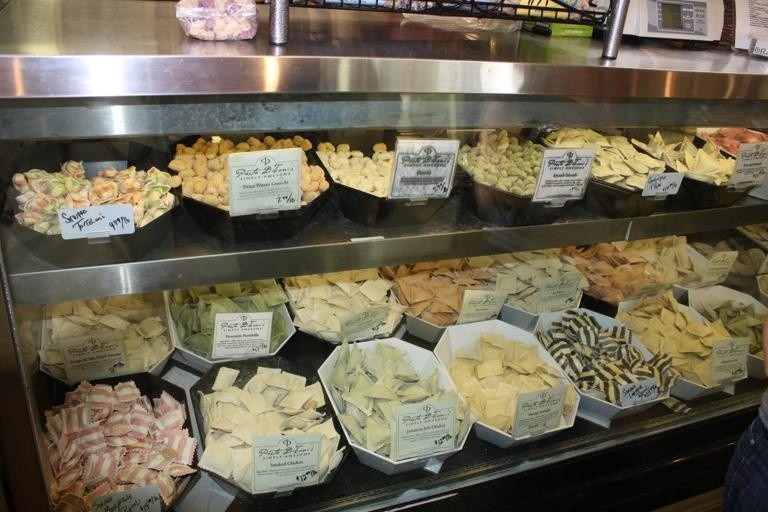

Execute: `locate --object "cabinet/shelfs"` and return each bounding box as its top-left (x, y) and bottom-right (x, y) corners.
top-left (0, 0), bottom-right (768, 511)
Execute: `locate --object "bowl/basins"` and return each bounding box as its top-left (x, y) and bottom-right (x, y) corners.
top-left (9, 131), bottom-right (767, 509)
